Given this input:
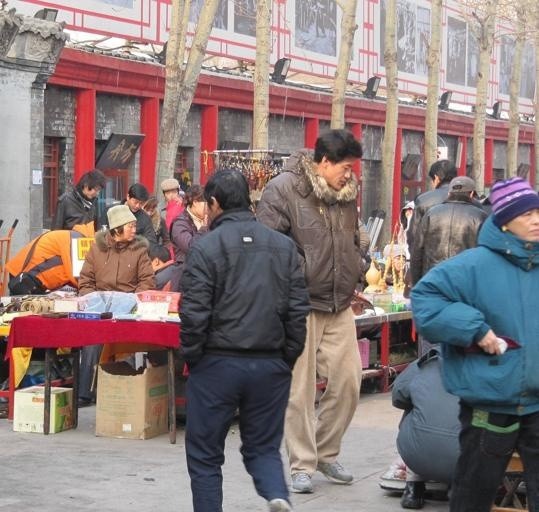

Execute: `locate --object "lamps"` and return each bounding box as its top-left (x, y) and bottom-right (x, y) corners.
top-left (361, 76), bottom-right (381, 99)
top-left (438, 91), bottom-right (452, 110)
top-left (34, 8), bottom-right (58, 21)
top-left (492, 101), bottom-right (502, 120)
top-left (269, 57), bottom-right (291, 84)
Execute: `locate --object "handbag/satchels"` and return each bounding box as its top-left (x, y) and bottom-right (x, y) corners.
top-left (9, 272), bottom-right (48, 294)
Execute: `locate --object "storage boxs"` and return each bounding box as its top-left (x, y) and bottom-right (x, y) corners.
top-left (96, 360), bottom-right (168, 439)
top-left (12, 385), bottom-right (78, 433)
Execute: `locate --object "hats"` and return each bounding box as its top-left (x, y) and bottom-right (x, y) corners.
top-left (107, 205), bottom-right (137, 230)
top-left (489, 177), bottom-right (539, 227)
top-left (449, 176), bottom-right (476, 192)
top-left (161, 179), bottom-right (178, 191)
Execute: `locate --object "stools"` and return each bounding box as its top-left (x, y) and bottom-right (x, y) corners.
top-left (489, 456), bottom-right (531, 512)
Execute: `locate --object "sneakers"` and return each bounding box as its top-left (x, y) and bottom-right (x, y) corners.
top-left (401, 481), bottom-right (424, 508)
top-left (268, 498), bottom-right (291, 511)
top-left (317, 461), bottom-right (353, 484)
top-left (291, 473), bottom-right (313, 493)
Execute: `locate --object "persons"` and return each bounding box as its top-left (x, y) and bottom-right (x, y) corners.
top-left (357, 161), bottom-right (490, 357)
top-left (254, 128), bottom-right (371, 496)
top-left (391, 340), bottom-right (462, 510)
top-left (177, 167), bottom-right (310, 510)
top-left (6, 170), bottom-right (213, 406)
top-left (409, 174), bottom-right (539, 512)
